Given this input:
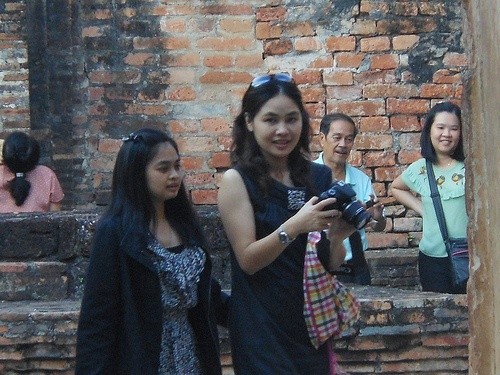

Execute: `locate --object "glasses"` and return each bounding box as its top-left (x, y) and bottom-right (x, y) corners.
top-left (251, 73), bottom-right (293, 88)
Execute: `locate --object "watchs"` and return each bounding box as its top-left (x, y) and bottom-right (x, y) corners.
top-left (277, 226), bottom-right (296, 245)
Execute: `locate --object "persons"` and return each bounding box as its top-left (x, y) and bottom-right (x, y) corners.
top-left (310, 112), bottom-right (386, 286)
top-left (75, 128), bottom-right (231, 375)
top-left (390, 101), bottom-right (470, 294)
top-left (216, 72), bottom-right (367, 375)
top-left (0, 130), bottom-right (64, 212)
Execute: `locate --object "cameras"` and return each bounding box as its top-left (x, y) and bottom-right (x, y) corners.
top-left (318, 180), bottom-right (372, 230)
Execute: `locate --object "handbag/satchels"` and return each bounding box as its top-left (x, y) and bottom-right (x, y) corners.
top-left (445, 237), bottom-right (469, 284)
top-left (303, 231), bottom-right (359, 349)
top-left (338, 258), bottom-right (371, 284)
